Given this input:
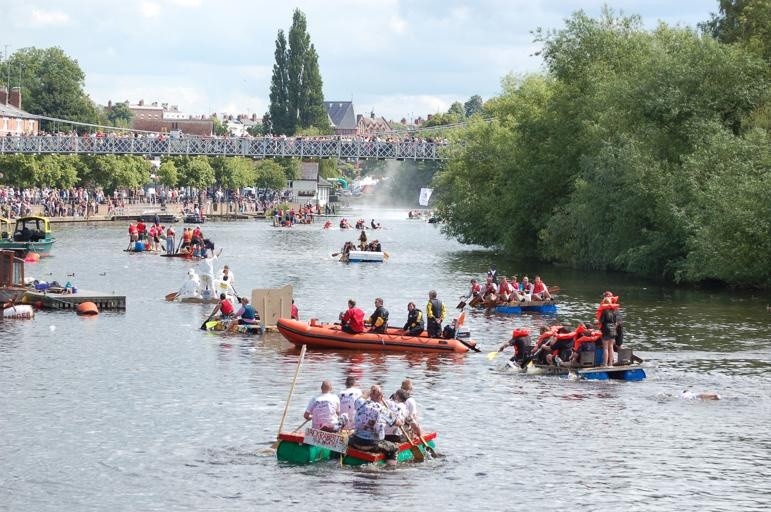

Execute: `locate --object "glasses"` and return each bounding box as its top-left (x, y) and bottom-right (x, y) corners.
top-left (375, 301), bottom-right (380, 303)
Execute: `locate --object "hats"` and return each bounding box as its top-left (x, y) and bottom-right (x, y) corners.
top-left (602, 291), bottom-right (613, 297)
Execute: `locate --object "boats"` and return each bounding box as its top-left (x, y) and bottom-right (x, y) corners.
top-left (183, 213), bottom-right (204, 223)
top-left (486, 351), bottom-right (645, 381)
top-left (276, 430), bottom-right (437, 469)
top-left (275, 312), bottom-right (477, 353)
top-left (180, 298), bottom-right (232, 303)
top-left (339, 250), bottom-right (388, 263)
top-left (160, 253), bottom-right (217, 258)
top-left (123, 247), bottom-right (169, 253)
top-left (473, 300), bottom-right (557, 314)
top-left (0, 216), bottom-right (56, 258)
top-left (204, 311), bottom-right (279, 335)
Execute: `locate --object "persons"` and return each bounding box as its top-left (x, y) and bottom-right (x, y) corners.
top-left (494, 328), bottom-right (531, 370)
top-left (531, 274), bottom-right (554, 302)
top-left (408, 208), bottom-right (441, 220)
top-left (175, 268), bottom-right (202, 300)
top-left (333, 229), bottom-right (390, 265)
top-left (216, 274), bottom-right (235, 300)
top-left (198, 248), bottom-right (223, 299)
top-left (218, 270), bottom-right (229, 279)
top-left (527, 326), bottom-right (551, 365)
top-left (568, 330), bottom-right (597, 367)
top-left (1, 184), bottom-right (336, 229)
top-left (339, 216), bottom-right (382, 231)
top-left (202, 293), bottom-right (236, 331)
top-left (339, 299), bottom-right (364, 334)
top-left (116, 212), bottom-right (221, 259)
top-left (397, 379), bottom-right (422, 438)
top-left (303, 380), bottom-right (349, 433)
top-left (460, 279), bottom-right (483, 301)
top-left (339, 376), bottom-right (362, 430)
top-left (347, 384), bottom-right (398, 467)
top-left (0, 133), bottom-right (471, 161)
top-left (479, 279), bottom-right (498, 305)
top-left (219, 297), bottom-right (261, 335)
top-left (364, 297), bottom-right (388, 333)
top-left (289, 299), bottom-right (299, 320)
top-left (424, 290), bottom-right (447, 337)
top-left (541, 325), bottom-right (575, 365)
top-left (221, 265), bottom-right (233, 276)
top-left (0, 224), bottom-right (46, 244)
top-left (495, 275), bottom-right (526, 304)
top-left (575, 317), bottom-right (601, 336)
top-left (380, 389), bottom-right (411, 443)
top-left (400, 301), bottom-right (424, 336)
top-left (507, 276), bottom-right (520, 293)
top-left (518, 275), bottom-right (534, 302)
top-left (596, 299), bottom-right (617, 369)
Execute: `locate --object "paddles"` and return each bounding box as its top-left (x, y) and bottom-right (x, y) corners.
top-left (381, 249), bottom-right (390, 258)
top-left (230, 283), bottom-right (243, 304)
top-left (488, 343), bottom-right (511, 360)
top-left (380, 397), bottom-right (425, 463)
top-left (546, 285), bottom-right (561, 295)
top-left (175, 236), bottom-right (182, 254)
top-left (164, 293), bottom-right (181, 303)
top-left (633, 352), bottom-right (644, 364)
top-left (165, 290), bottom-right (180, 299)
top-left (159, 242), bottom-right (166, 252)
top-left (332, 251), bottom-right (343, 258)
top-left (270, 417), bottom-right (313, 450)
top-left (457, 292), bottom-right (472, 309)
top-left (201, 305), bottom-right (219, 330)
top-left (233, 293), bottom-right (242, 301)
top-left (123, 242), bottom-right (130, 252)
top-left (418, 432), bottom-right (438, 459)
top-left (520, 335), bottom-right (555, 368)
